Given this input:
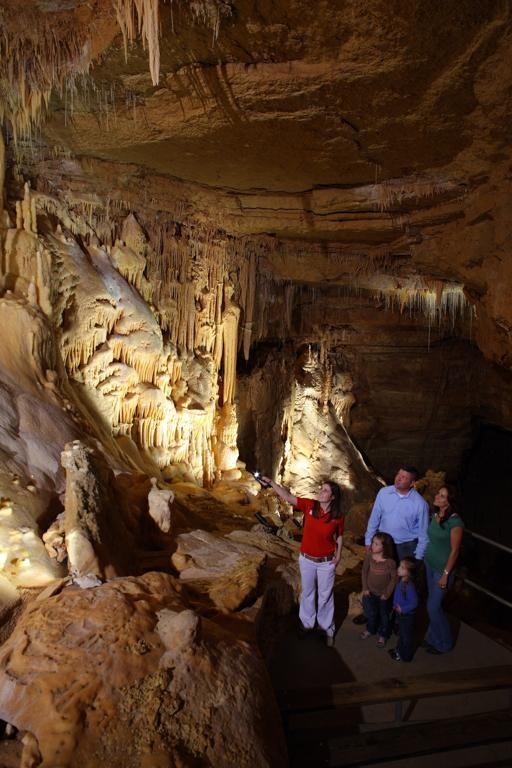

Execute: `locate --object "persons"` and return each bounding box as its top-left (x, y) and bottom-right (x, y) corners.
top-left (352, 465), bottom-right (429, 637)
top-left (359, 531), bottom-right (400, 649)
top-left (387, 556), bottom-right (421, 662)
top-left (421, 485), bottom-right (468, 655)
top-left (256, 475), bottom-right (346, 648)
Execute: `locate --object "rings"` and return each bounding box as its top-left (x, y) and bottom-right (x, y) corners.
top-left (441, 585), bottom-right (444, 587)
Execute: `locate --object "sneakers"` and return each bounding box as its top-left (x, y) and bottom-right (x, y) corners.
top-left (325, 636), bottom-right (333, 647)
top-left (353, 614), bottom-right (400, 648)
top-left (389, 647), bottom-right (404, 662)
top-left (419, 640), bottom-right (443, 654)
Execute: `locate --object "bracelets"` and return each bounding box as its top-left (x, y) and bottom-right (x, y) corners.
top-left (443, 568), bottom-right (449, 577)
top-left (267, 479), bottom-right (272, 484)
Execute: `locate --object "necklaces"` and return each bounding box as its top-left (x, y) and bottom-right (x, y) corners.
top-left (436, 513), bottom-right (442, 520)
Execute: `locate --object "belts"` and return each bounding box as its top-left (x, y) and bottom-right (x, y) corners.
top-left (300, 552), bottom-right (332, 563)
